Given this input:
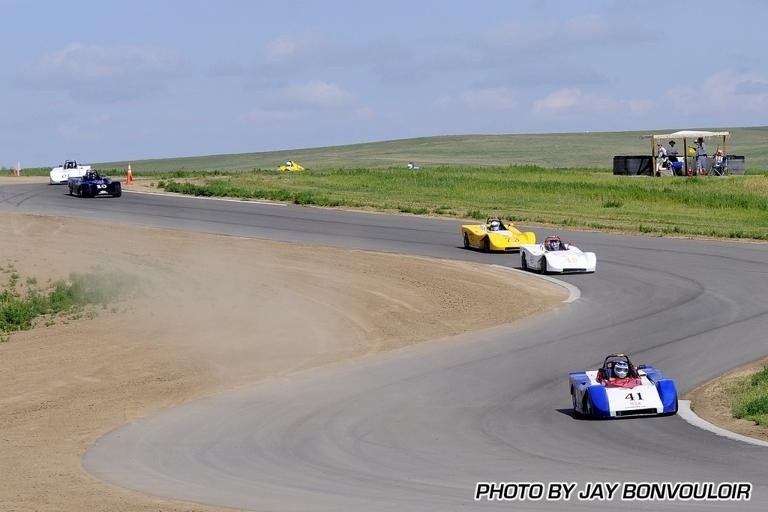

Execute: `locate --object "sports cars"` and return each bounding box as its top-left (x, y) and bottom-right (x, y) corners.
top-left (68, 170), bottom-right (121, 199)
top-left (520, 237), bottom-right (597, 274)
top-left (462, 218), bottom-right (536, 252)
top-left (277, 159), bottom-right (305, 172)
top-left (569, 353), bottom-right (678, 418)
top-left (50, 160), bottom-right (92, 185)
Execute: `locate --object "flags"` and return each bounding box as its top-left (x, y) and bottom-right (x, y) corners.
top-left (688, 147), bottom-right (696, 156)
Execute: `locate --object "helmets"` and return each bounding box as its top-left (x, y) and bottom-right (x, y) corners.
top-left (491, 221), bottom-right (500, 231)
top-left (545, 236), bottom-right (560, 252)
top-left (613, 360), bottom-right (628, 378)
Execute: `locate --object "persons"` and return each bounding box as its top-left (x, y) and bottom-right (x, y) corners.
top-left (656, 143), bottom-right (667, 158)
top-left (666, 140), bottom-right (679, 154)
top-left (712, 149), bottom-right (723, 167)
top-left (695, 138), bottom-right (707, 176)
top-left (694, 140), bottom-right (700, 148)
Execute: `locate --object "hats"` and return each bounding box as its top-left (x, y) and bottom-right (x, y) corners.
top-left (668, 140), bottom-right (675, 144)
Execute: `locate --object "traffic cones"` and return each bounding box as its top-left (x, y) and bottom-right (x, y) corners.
top-left (124, 162), bottom-right (133, 185)
top-left (12, 168), bottom-right (17, 177)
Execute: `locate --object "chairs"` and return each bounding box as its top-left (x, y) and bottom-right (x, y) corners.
top-left (708, 156), bottom-right (727, 177)
